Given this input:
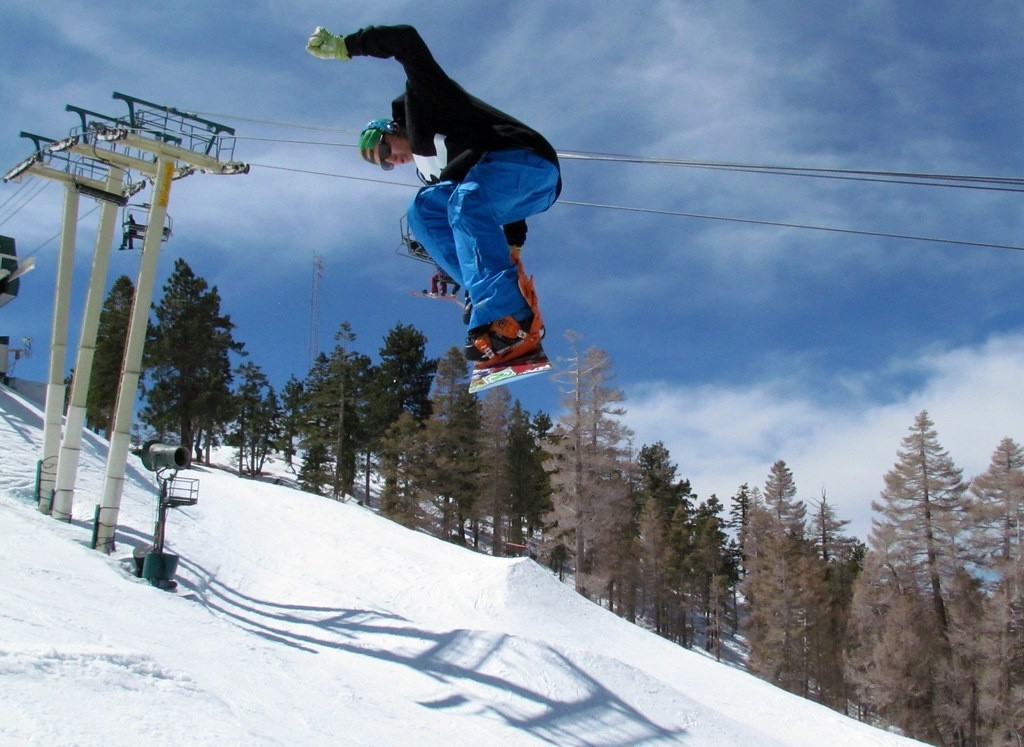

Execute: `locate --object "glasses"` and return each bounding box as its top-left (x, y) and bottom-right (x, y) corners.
top-left (378, 134), bottom-right (395, 171)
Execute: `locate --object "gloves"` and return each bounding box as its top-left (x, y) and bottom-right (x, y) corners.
top-left (304, 25), bottom-right (350, 61)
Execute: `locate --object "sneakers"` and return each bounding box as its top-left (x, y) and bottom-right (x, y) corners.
top-left (464, 314), bottom-right (546, 361)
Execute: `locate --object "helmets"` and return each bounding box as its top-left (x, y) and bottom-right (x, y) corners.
top-left (359, 118), bottom-right (396, 166)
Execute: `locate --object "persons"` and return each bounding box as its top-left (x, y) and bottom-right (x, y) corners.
top-left (119, 214), bottom-right (136, 250)
top-left (305, 24), bottom-right (562, 394)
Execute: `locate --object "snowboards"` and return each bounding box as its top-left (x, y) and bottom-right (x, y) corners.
top-left (467, 246), bottom-right (552, 393)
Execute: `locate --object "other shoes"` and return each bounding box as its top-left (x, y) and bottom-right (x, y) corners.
top-left (440, 291), bottom-right (447, 296)
top-left (430, 292), bottom-right (439, 297)
top-left (450, 294), bottom-right (457, 299)
